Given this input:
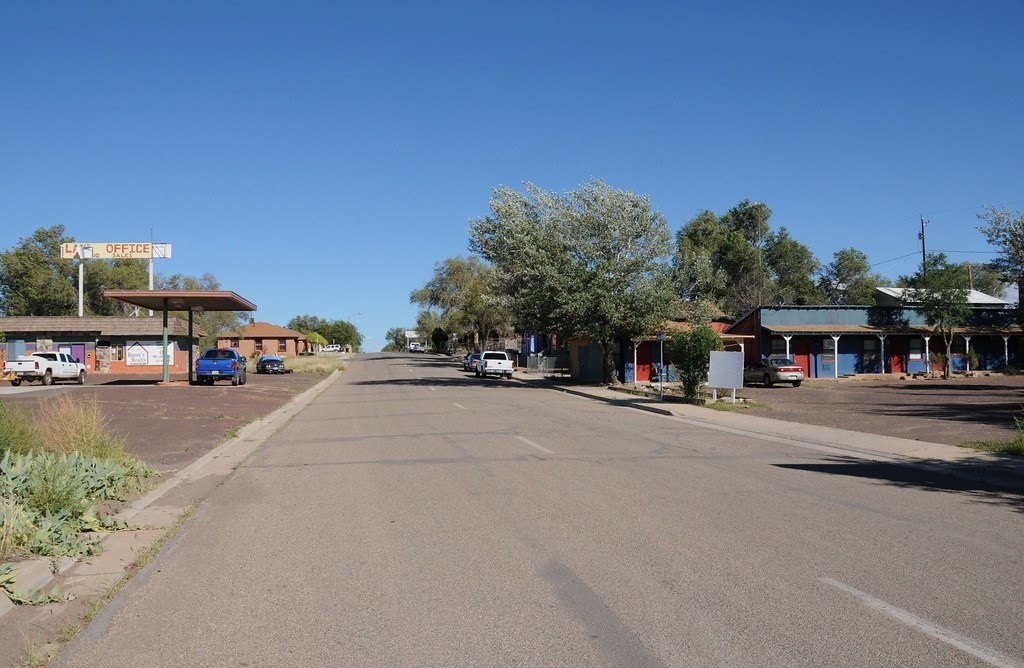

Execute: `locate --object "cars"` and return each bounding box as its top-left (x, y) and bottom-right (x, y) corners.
top-left (256, 356), bottom-right (285, 374)
top-left (464, 354), bottom-right (481, 372)
top-left (744, 358), bottom-right (805, 388)
top-left (409, 342), bottom-right (425, 354)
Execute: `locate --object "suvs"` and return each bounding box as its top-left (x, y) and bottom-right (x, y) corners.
top-left (321, 344), bottom-right (342, 352)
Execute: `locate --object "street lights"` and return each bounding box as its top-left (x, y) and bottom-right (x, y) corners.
top-left (347, 312), bottom-right (362, 323)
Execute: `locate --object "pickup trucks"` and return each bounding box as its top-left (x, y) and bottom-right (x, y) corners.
top-left (3, 351), bottom-right (87, 386)
top-left (476, 351), bottom-right (515, 379)
top-left (195, 347), bottom-right (247, 386)
